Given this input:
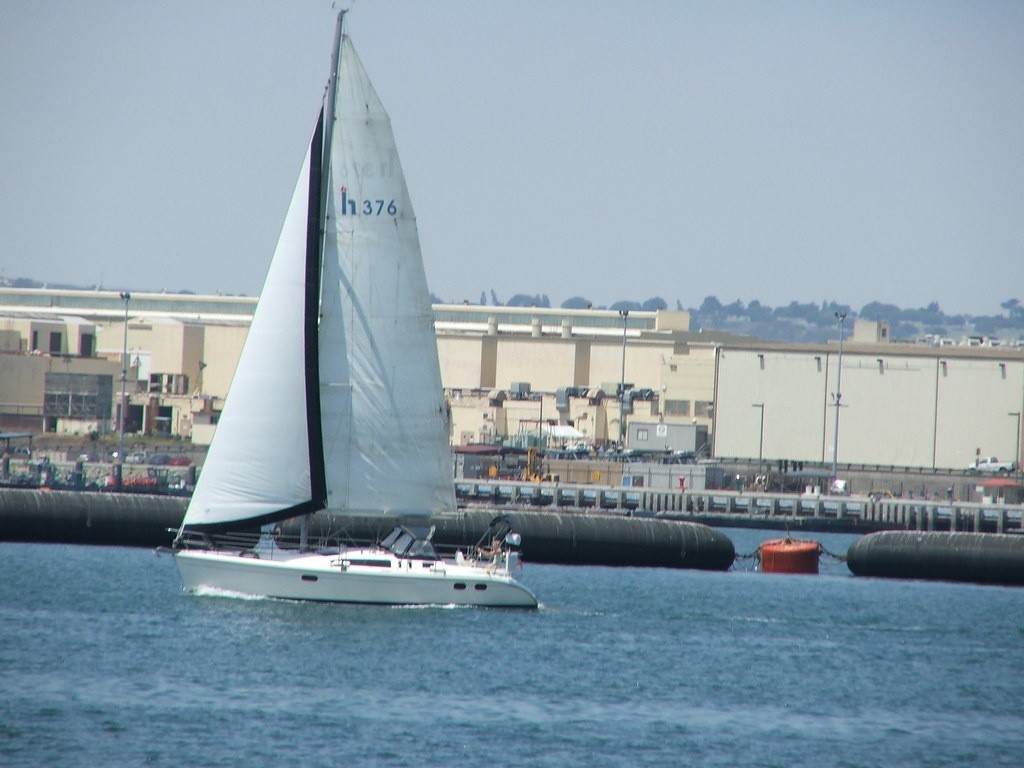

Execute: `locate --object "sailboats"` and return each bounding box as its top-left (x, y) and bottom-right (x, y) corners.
top-left (154, 8), bottom-right (539, 606)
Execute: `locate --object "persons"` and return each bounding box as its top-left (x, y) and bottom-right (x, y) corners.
top-left (476, 540), bottom-right (502, 568)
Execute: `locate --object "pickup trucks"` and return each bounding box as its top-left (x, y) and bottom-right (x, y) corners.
top-left (968, 456), bottom-right (1015, 478)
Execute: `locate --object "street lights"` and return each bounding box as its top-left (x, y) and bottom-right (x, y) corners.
top-left (1008, 412), bottom-right (1020, 483)
top-left (618, 307), bottom-right (629, 446)
top-left (118, 292), bottom-right (131, 457)
top-left (751, 401), bottom-right (765, 475)
top-left (832, 310), bottom-right (848, 477)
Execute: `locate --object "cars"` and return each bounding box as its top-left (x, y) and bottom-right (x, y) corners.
top-left (124, 450), bottom-right (192, 465)
